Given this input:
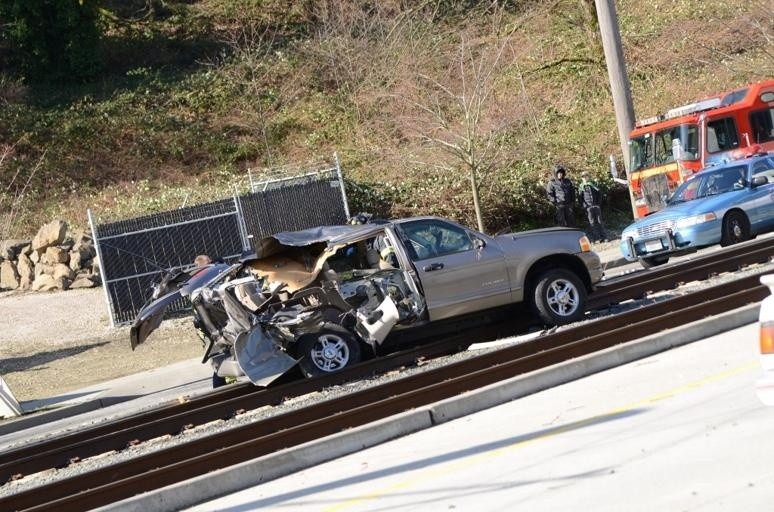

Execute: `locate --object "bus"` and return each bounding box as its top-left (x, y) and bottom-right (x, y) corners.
top-left (609, 78), bottom-right (774, 219)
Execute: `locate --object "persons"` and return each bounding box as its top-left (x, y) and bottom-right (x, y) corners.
top-left (193, 254), bottom-right (227, 389)
top-left (546, 165), bottom-right (610, 244)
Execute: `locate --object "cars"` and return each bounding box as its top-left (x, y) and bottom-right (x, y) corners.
top-left (619, 149), bottom-right (774, 269)
top-left (130, 151), bottom-right (607, 387)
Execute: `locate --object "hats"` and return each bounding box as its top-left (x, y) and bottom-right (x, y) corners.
top-left (580, 171), bottom-right (590, 177)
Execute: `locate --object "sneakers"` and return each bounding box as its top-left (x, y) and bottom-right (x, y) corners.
top-left (595, 238), bottom-right (610, 244)
